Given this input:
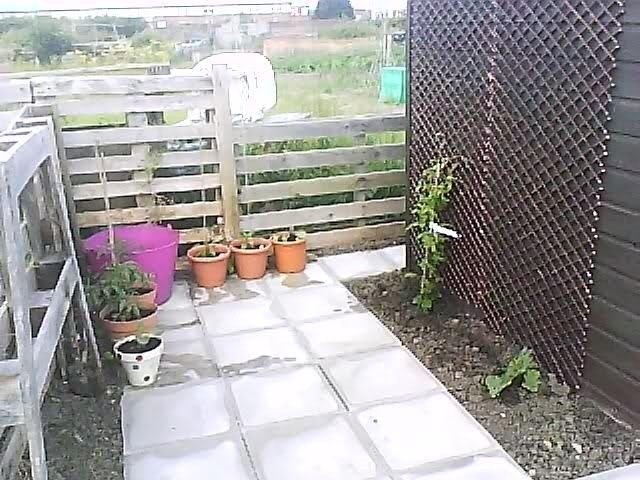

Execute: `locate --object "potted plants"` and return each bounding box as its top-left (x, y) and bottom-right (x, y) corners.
top-left (91, 259), bottom-right (167, 387)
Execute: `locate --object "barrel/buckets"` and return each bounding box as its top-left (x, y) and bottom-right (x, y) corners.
top-left (83, 224), bottom-right (180, 305)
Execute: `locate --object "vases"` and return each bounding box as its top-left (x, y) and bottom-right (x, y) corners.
top-left (185, 225), bottom-right (307, 287)
